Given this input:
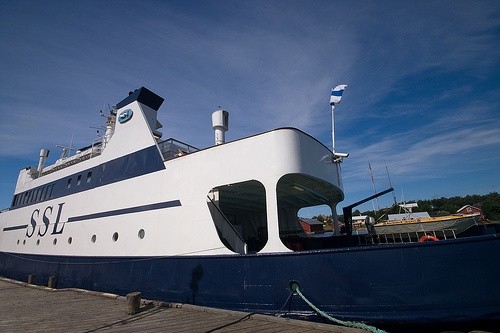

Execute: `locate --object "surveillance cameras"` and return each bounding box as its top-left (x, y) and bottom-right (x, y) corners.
top-left (334, 152), bottom-right (349, 158)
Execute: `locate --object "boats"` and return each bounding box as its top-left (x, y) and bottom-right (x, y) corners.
top-left (1, 85), bottom-right (500, 325)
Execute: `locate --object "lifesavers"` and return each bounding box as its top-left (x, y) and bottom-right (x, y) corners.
top-left (419, 235), bottom-right (438, 242)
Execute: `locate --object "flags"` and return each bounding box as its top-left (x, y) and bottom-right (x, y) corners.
top-left (330, 84), bottom-right (349, 106)
